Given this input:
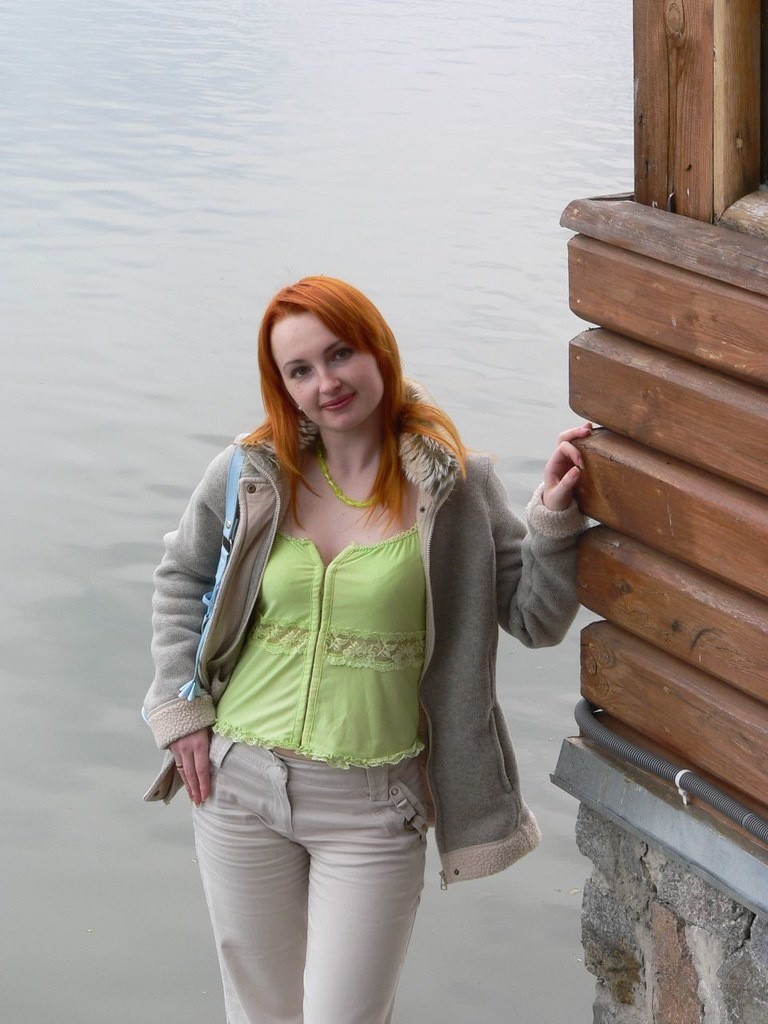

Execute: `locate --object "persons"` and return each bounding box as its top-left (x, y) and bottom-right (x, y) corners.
top-left (142, 277), bottom-right (593, 1024)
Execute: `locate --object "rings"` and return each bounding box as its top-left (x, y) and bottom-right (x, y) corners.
top-left (175, 765), bottom-right (183, 768)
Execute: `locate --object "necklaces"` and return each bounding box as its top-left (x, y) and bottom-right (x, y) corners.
top-left (312, 431), bottom-right (393, 507)
